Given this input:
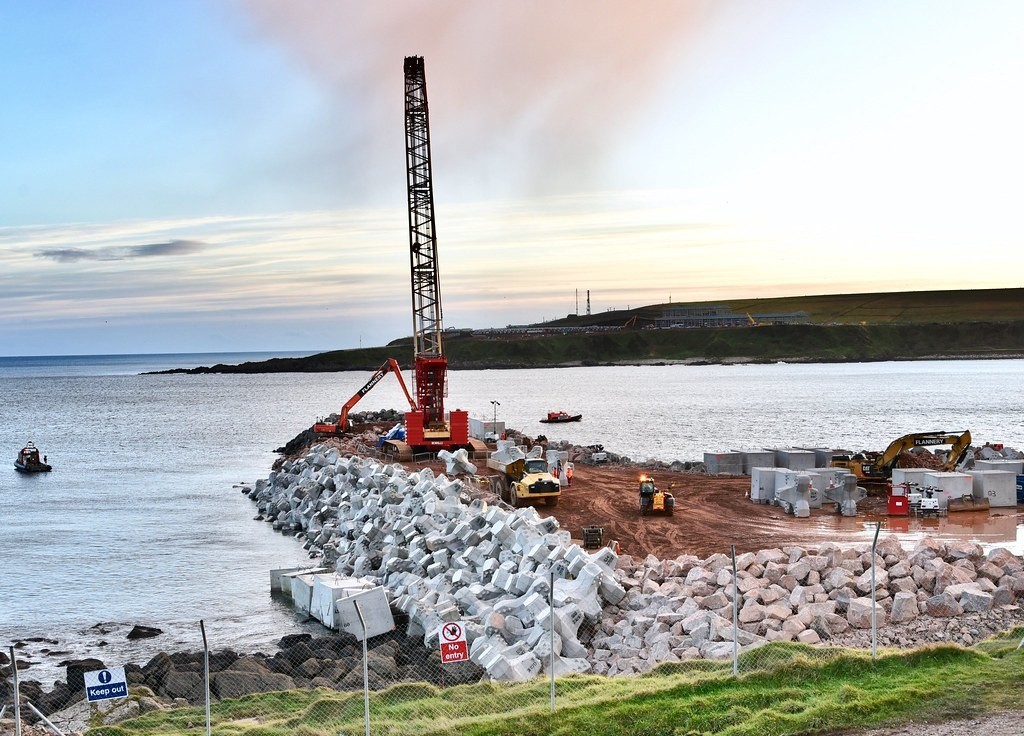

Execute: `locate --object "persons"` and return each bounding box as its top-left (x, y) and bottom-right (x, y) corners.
top-left (566, 467), bottom-right (573, 487)
top-left (551, 468), bottom-right (557, 478)
top-left (501, 432), bottom-right (505, 440)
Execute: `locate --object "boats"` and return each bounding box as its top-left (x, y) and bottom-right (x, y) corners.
top-left (14, 442), bottom-right (52, 473)
top-left (539, 411), bottom-right (583, 423)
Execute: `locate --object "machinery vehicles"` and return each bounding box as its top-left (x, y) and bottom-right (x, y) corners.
top-left (312, 357), bottom-right (418, 438)
top-left (485, 451), bottom-right (561, 507)
top-left (830, 430), bottom-right (972, 485)
top-left (380, 54), bottom-right (490, 464)
top-left (641, 477), bottom-right (675, 515)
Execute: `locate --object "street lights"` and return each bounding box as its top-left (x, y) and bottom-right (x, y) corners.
top-left (490, 400), bottom-right (500, 434)
